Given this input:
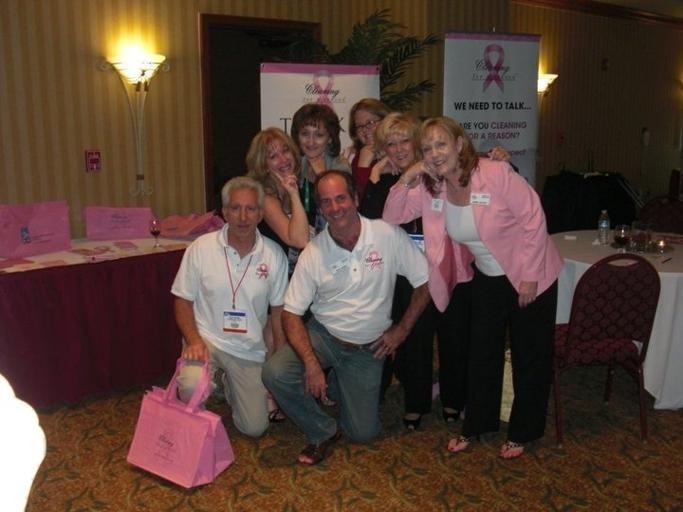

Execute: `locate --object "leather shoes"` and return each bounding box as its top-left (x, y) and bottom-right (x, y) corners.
top-left (403, 411), bottom-right (421, 432)
top-left (442, 404), bottom-right (461, 423)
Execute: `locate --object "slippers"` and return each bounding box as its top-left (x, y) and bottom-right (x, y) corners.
top-left (497, 440), bottom-right (529, 459)
top-left (445, 434), bottom-right (476, 453)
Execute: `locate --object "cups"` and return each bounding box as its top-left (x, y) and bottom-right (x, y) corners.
top-left (652, 236), bottom-right (671, 251)
top-left (630, 221), bottom-right (642, 251)
top-left (638, 223), bottom-right (655, 253)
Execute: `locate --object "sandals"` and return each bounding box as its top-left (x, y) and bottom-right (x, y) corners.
top-left (296, 432), bottom-right (341, 465)
top-left (267, 394), bottom-right (286, 423)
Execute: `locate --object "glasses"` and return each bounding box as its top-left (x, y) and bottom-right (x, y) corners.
top-left (352, 119), bottom-right (382, 133)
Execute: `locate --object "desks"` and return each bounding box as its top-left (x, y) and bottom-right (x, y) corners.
top-left (0, 234), bottom-right (196, 409)
top-left (547, 229), bottom-right (683, 411)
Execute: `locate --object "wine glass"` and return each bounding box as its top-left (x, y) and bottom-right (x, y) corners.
top-left (149, 220), bottom-right (162, 248)
top-left (613, 225), bottom-right (629, 253)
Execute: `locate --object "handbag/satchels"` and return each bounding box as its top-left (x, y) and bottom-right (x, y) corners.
top-left (127, 385), bottom-right (235, 488)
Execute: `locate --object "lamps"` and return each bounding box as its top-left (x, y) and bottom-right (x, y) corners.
top-left (99, 52), bottom-right (169, 195)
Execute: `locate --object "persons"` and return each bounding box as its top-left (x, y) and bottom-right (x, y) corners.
top-left (166, 98), bottom-right (563, 457)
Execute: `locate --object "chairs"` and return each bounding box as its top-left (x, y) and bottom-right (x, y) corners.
top-left (669, 169), bottom-right (680, 199)
top-left (552, 252), bottom-right (661, 450)
top-left (638, 195), bottom-right (683, 235)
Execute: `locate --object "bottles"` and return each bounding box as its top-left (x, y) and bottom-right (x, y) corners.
top-left (598, 209), bottom-right (610, 245)
